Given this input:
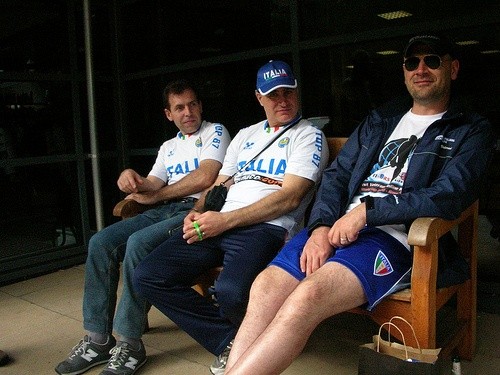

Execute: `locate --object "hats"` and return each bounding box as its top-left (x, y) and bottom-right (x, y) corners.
top-left (256, 60), bottom-right (298, 96)
top-left (403, 32), bottom-right (455, 57)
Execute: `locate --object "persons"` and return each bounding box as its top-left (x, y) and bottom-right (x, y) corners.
top-left (55, 80), bottom-right (232, 375)
top-left (221, 34), bottom-right (498, 375)
top-left (131, 59), bottom-right (329, 375)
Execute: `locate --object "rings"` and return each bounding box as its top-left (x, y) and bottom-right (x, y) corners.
top-left (202, 231), bottom-right (206, 236)
top-left (340, 239), bottom-right (347, 242)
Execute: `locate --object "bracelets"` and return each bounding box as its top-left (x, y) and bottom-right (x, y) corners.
top-left (191, 209), bottom-right (203, 215)
top-left (194, 220), bottom-right (203, 241)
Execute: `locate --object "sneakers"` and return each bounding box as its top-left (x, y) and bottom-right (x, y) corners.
top-left (98, 340), bottom-right (147, 375)
top-left (209, 339), bottom-right (235, 375)
top-left (55, 334), bottom-right (116, 375)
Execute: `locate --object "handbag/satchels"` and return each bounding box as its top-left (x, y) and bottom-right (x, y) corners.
top-left (358, 315), bottom-right (442, 375)
top-left (204, 182), bottom-right (228, 212)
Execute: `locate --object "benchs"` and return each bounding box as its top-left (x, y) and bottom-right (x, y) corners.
top-left (112, 137), bottom-right (479, 364)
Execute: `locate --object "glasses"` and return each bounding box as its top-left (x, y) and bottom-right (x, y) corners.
top-left (403, 55), bottom-right (454, 71)
